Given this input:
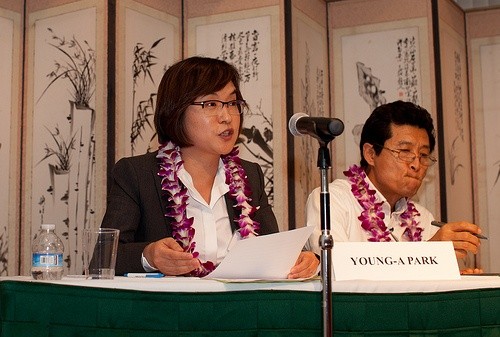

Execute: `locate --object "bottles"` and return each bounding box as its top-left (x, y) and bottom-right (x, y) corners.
top-left (31, 224), bottom-right (65, 281)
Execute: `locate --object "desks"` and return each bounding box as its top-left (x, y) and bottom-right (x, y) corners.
top-left (0, 274), bottom-right (500, 337)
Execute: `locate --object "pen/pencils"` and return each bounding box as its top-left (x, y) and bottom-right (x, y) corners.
top-left (431, 220), bottom-right (489, 240)
top-left (123, 272), bottom-right (164, 278)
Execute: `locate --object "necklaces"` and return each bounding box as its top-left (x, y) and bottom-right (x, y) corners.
top-left (157, 141), bottom-right (260, 277)
top-left (343, 162), bottom-right (424, 241)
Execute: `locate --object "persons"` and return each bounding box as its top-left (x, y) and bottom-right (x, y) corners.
top-left (83, 56), bottom-right (321, 279)
top-left (305, 98), bottom-right (485, 273)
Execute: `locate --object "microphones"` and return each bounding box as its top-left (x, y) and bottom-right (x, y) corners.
top-left (289, 112), bottom-right (344, 137)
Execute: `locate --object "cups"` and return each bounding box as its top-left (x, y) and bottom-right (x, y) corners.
top-left (83, 228), bottom-right (120, 279)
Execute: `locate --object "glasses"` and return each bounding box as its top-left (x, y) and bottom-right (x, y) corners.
top-left (375, 143), bottom-right (437, 166)
top-left (191, 100), bottom-right (246, 115)
